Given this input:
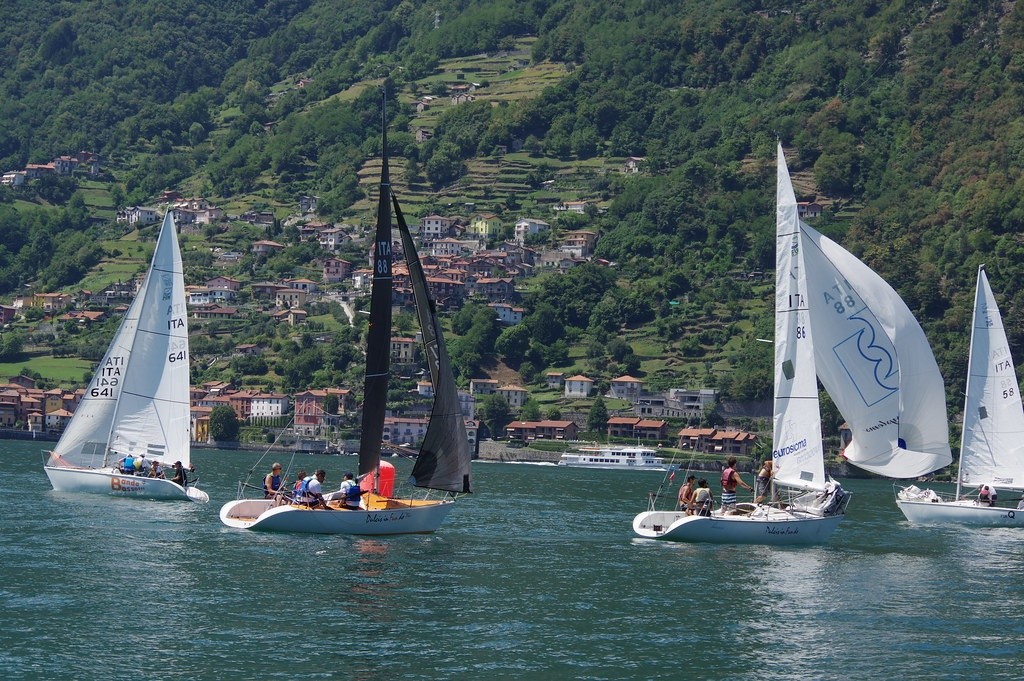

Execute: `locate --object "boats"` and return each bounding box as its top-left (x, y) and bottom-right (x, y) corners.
top-left (556, 439), bottom-right (682, 473)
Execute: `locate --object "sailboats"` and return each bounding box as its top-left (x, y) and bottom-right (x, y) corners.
top-left (802, 215), bottom-right (1024, 528)
top-left (217, 89), bottom-right (477, 537)
top-left (39, 203), bottom-right (210, 505)
top-left (628, 135), bottom-right (855, 545)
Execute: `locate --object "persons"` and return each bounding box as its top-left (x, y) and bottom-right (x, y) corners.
top-left (291, 469), bottom-right (334, 510)
top-left (118, 453), bottom-right (166, 479)
top-left (262, 462), bottom-right (291, 508)
top-left (677, 475), bottom-right (715, 517)
top-left (170, 461), bottom-right (188, 487)
top-left (976, 484), bottom-right (997, 507)
top-left (719, 456), bottom-right (755, 517)
top-left (336, 472), bottom-right (360, 511)
top-left (753, 460), bottom-right (772, 509)
top-left (1015, 495), bottom-right (1024, 510)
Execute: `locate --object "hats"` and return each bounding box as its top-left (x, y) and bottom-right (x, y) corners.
top-left (688, 474), bottom-right (696, 480)
top-left (272, 463), bottom-right (280, 468)
top-left (152, 461), bottom-right (159, 464)
top-left (342, 471), bottom-right (353, 478)
top-left (139, 453), bottom-right (146, 456)
top-left (174, 461), bottom-right (181, 464)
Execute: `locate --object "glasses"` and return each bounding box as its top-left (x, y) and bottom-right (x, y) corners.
top-left (278, 467), bottom-right (282, 470)
